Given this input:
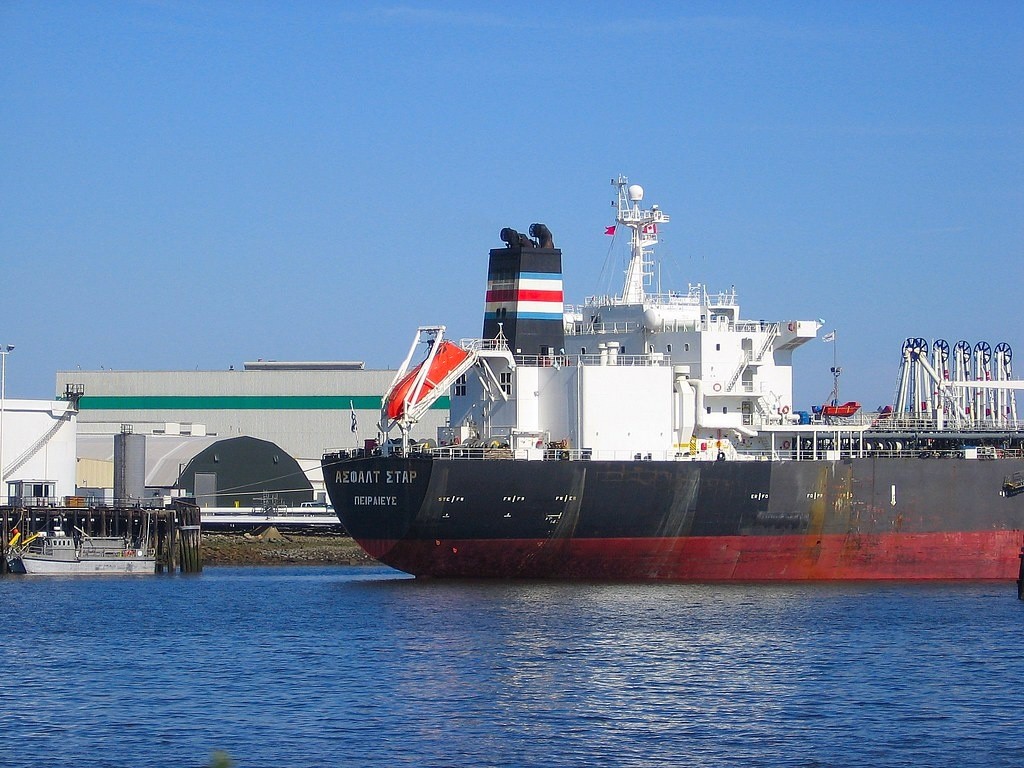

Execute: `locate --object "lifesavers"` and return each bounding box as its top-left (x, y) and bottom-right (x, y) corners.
top-left (782, 405), bottom-right (789, 414)
top-left (783, 441), bottom-right (790, 449)
top-left (717, 452), bottom-right (725, 462)
top-left (788, 323), bottom-right (795, 332)
top-left (713, 383), bottom-right (722, 392)
top-left (454, 437), bottom-right (460, 444)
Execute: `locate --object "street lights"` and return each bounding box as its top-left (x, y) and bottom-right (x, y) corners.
top-left (0, 344), bottom-right (16, 510)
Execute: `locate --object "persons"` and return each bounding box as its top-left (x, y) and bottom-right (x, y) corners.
top-left (561, 438), bottom-right (570, 459)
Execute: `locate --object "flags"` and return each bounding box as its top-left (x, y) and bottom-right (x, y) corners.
top-left (604, 226), bottom-right (615, 235)
top-left (822, 332), bottom-right (834, 342)
top-left (644, 225), bottom-right (656, 234)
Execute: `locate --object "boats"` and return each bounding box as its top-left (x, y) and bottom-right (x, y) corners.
top-left (19, 524), bottom-right (158, 576)
top-left (317, 170), bottom-right (1023, 586)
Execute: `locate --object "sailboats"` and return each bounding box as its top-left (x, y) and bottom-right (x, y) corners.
top-left (820, 328), bottom-right (861, 418)
